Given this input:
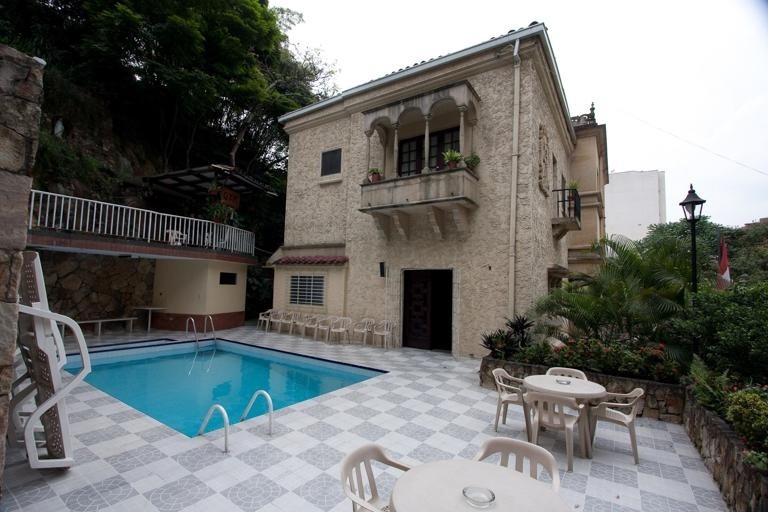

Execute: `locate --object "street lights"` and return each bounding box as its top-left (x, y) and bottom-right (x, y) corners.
top-left (680, 182), bottom-right (707, 293)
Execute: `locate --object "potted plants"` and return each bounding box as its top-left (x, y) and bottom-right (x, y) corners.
top-left (204, 202), bottom-right (246, 228)
top-left (206, 181), bottom-right (221, 195)
top-left (367, 167), bottom-right (384, 184)
top-left (441, 150), bottom-right (465, 170)
top-left (569, 179), bottom-right (581, 208)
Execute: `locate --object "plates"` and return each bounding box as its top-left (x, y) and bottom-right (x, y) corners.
top-left (462, 484), bottom-right (496, 507)
top-left (556, 378), bottom-right (571, 385)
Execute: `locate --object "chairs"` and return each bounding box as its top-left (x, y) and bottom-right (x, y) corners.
top-left (491, 369), bottom-right (534, 443)
top-left (257, 308), bottom-right (393, 350)
top-left (546, 367), bottom-right (588, 381)
top-left (522, 391), bottom-right (586, 472)
top-left (167, 229), bottom-right (188, 248)
top-left (477, 437), bottom-right (560, 488)
top-left (214, 228), bottom-right (229, 249)
top-left (589, 387), bottom-right (645, 465)
top-left (340, 444), bottom-right (409, 512)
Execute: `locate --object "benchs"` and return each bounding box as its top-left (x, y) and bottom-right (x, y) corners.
top-left (57, 317), bottom-right (138, 340)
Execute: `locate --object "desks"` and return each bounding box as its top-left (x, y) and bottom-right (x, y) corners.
top-left (522, 375), bottom-right (607, 459)
top-left (391, 459), bottom-right (561, 512)
top-left (135, 307), bottom-right (167, 334)
